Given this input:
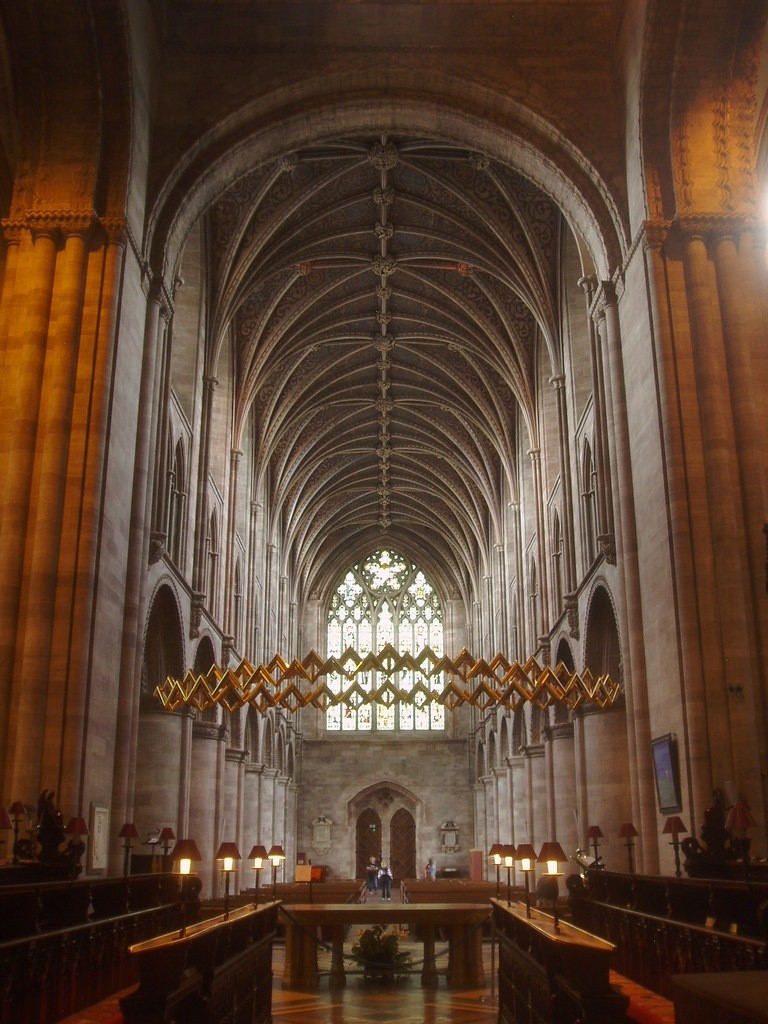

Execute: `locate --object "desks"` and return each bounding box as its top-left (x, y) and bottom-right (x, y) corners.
top-left (276, 902), bottom-right (494, 990)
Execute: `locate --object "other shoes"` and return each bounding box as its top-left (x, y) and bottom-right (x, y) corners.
top-left (369, 892), bottom-right (372, 895)
top-left (387, 898), bottom-right (390, 901)
top-left (382, 898), bottom-right (385, 900)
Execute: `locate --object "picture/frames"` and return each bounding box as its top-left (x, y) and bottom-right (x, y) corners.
top-left (650, 733), bottom-right (683, 813)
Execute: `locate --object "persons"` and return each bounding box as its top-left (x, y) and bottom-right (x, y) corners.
top-left (424, 859), bottom-right (436, 882)
top-left (365, 856), bottom-right (379, 896)
top-left (377, 862), bottom-right (393, 901)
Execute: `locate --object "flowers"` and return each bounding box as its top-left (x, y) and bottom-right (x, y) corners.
top-left (350, 923), bottom-right (413, 976)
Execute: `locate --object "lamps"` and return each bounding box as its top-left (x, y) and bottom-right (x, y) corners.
top-left (9, 801), bottom-right (26, 823)
top-left (168, 840), bottom-right (201, 879)
top-left (618, 823), bottom-right (640, 847)
top-left (490, 843), bottom-right (503, 865)
top-left (215, 843), bottom-right (239, 872)
top-left (725, 806), bottom-right (758, 848)
top-left (661, 817), bottom-right (687, 846)
top-left (159, 827), bottom-right (174, 849)
top-left (247, 846), bottom-right (268, 870)
top-left (64, 816), bottom-right (88, 837)
top-left (502, 845), bottom-right (517, 870)
top-left (267, 846), bottom-right (286, 868)
top-left (536, 841), bottom-right (569, 877)
top-left (515, 845), bottom-right (538, 872)
top-left (587, 826), bottom-right (603, 847)
top-left (117, 822), bottom-right (138, 849)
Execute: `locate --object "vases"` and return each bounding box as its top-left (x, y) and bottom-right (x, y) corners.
top-left (364, 968), bottom-right (394, 983)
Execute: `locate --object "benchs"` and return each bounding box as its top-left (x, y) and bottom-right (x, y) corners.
top-left (399, 879), bottom-right (573, 942)
top-left (197, 881), bottom-right (367, 947)
